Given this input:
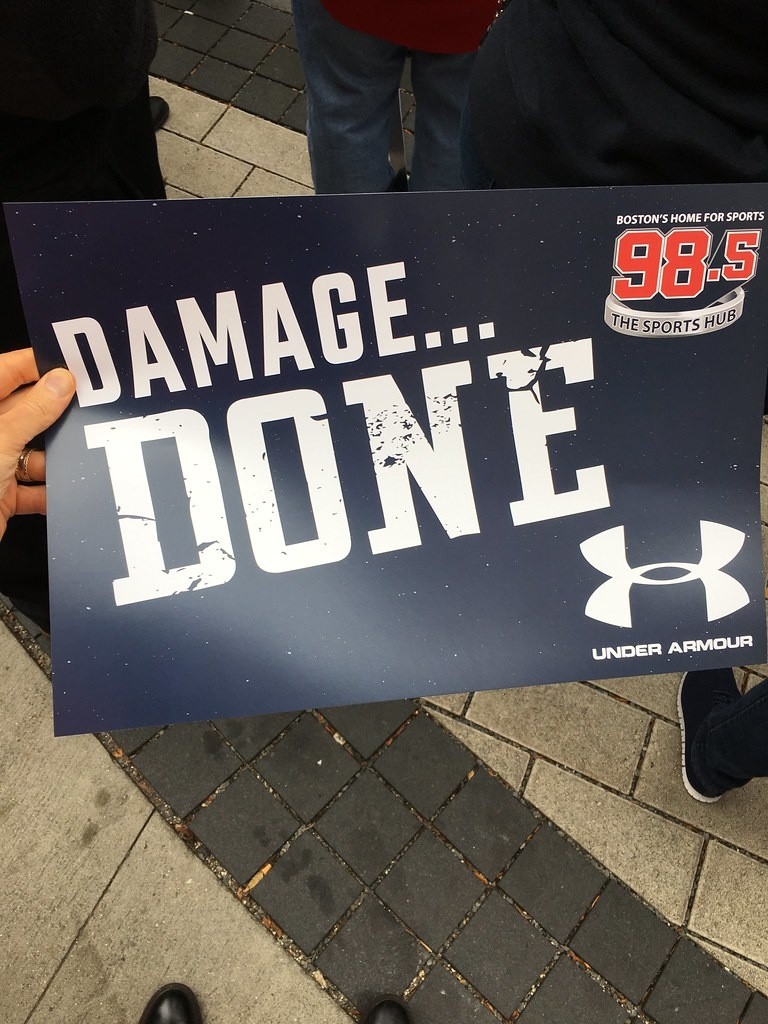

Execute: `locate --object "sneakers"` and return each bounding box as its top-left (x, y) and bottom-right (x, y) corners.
top-left (677, 668), bottom-right (743, 804)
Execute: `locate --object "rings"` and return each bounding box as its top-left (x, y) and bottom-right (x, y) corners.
top-left (15, 449), bottom-right (38, 482)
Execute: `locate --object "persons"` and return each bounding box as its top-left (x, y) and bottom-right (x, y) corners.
top-left (0, 344), bottom-right (76, 538)
top-left (678, 666), bottom-right (768, 803)
top-left (292, 0), bottom-right (499, 194)
top-left (459, 0), bottom-right (767, 189)
top-left (0, 1), bottom-right (170, 635)
top-left (139, 983), bottom-right (414, 1024)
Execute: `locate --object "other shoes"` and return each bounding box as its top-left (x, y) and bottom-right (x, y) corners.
top-left (149, 95), bottom-right (170, 129)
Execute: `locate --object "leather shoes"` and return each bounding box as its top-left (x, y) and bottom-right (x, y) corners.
top-left (362, 994), bottom-right (415, 1024)
top-left (137, 983), bottom-right (203, 1024)
